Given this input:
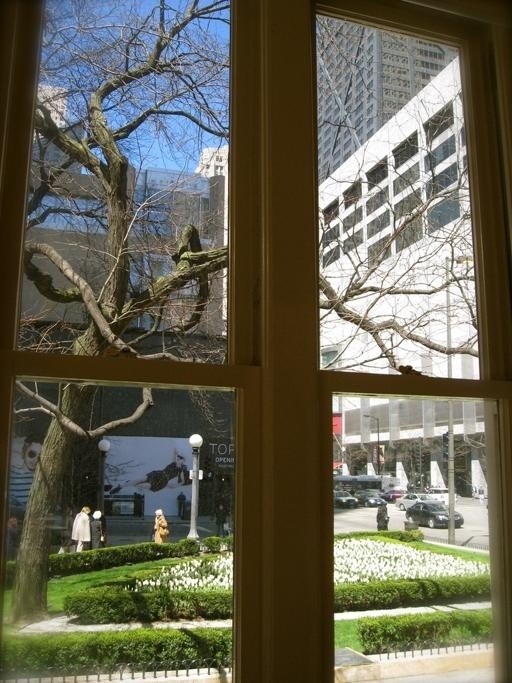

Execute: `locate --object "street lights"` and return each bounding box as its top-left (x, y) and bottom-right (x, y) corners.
top-left (185, 433), bottom-right (204, 542)
top-left (440, 255), bottom-right (464, 545)
top-left (362, 413), bottom-right (380, 474)
top-left (98, 438), bottom-right (110, 517)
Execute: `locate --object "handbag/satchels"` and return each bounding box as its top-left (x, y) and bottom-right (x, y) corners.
top-left (101, 536), bottom-right (105, 542)
top-left (160, 527), bottom-right (170, 536)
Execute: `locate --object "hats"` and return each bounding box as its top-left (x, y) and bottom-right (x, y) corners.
top-left (155, 509), bottom-right (162, 514)
top-left (93, 511), bottom-right (101, 519)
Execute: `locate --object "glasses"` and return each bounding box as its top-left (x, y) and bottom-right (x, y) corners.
top-left (178, 455), bottom-right (183, 459)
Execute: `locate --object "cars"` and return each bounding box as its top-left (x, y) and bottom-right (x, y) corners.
top-left (334, 487), bottom-right (463, 529)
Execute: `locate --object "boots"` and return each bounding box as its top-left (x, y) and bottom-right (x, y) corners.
top-left (104, 484), bottom-right (121, 494)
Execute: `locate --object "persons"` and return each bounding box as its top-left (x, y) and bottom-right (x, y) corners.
top-left (177, 490), bottom-right (186, 519)
top-left (9, 432), bottom-right (47, 512)
top-left (6, 517), bottom-right (22, 559)
top-left (375, 499), bottom-right (391, 531)
top-left (152, 509), bottom-right (169, 544)
top-left (108, 446), bottom-right (188, 494)
top-left (215, 501), bottom-right (226, 537)
top-left (70, 506), bottom-right (92, 551)
top-left (90, 509), bottom-right (108, 549)
top-left (90, 506), bottom-right (107, 534)
